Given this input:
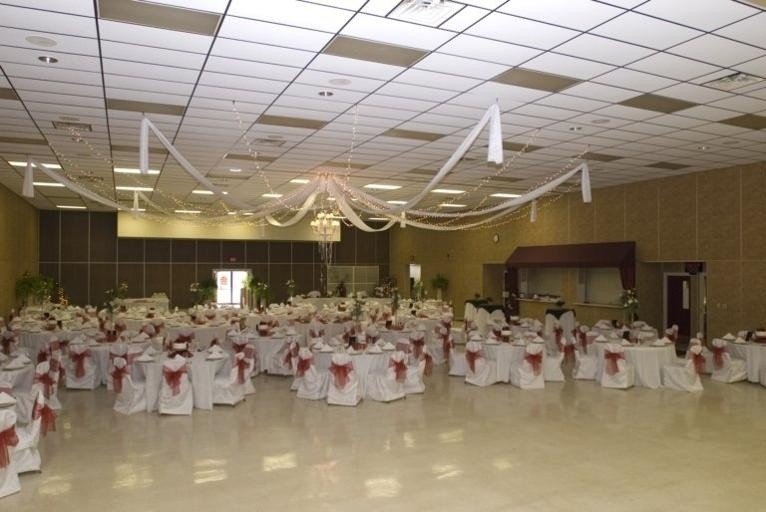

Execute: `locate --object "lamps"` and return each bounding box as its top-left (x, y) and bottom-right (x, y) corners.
top-left (311, 171), bottom-right (339, 244)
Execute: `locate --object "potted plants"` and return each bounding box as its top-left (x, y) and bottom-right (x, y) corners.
top-left (429, 273), bottom-right (448, 300)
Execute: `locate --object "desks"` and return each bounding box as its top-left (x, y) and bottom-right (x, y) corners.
top-left (721, 331), bottom-right (765, 387)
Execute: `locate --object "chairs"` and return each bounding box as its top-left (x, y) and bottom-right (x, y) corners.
top-left (448, 301), bottom-right (748, 391)
top-left (1, 338), bottom-right (66, 498)
top-left (9, 291), bottom-right (454, 416)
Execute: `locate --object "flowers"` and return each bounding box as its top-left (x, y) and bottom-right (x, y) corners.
top-left (244, 274), bottom-right (267, 291)
top-left (189, 276), bottom-right (218, 298)
top-left (118, 281), bottom-right (130, 292)
top-left (286, 278), bottom-right (295, 289)
